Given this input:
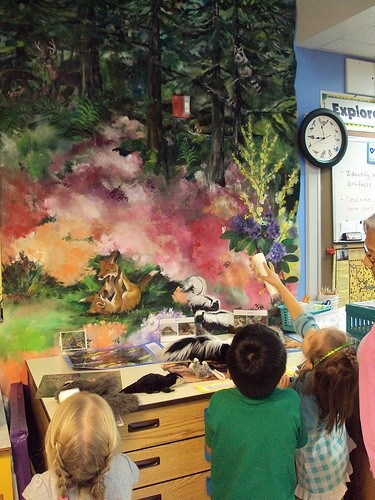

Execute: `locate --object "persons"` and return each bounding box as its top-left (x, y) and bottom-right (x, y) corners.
top-left (18, 390), bottom-right (141, 500)
top-left (261, 260), bottom-right (359, 500)
top-left (206, 323), bottom-right (308, 500)
top-left (354, 208), bottom-right (375, 498)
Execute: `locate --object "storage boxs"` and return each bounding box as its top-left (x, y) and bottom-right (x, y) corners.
top-left (278, 303), bottom-right (333, 332)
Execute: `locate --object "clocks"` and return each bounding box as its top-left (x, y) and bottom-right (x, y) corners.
top-left (298, 107), bottom-right (349, 169)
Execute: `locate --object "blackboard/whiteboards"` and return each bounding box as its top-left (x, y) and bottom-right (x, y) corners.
top-left (332, 136), bottom-right (375, 242)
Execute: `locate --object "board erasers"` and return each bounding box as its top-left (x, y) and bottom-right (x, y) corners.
top-left (344, 232), bottom-right (362, 241)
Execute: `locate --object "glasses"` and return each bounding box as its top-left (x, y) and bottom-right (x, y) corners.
top-left (362, 242), bottom-right (375, 268)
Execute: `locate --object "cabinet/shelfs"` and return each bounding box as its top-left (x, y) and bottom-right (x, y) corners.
top-left (24, 332), bottom-right (309, 500)
